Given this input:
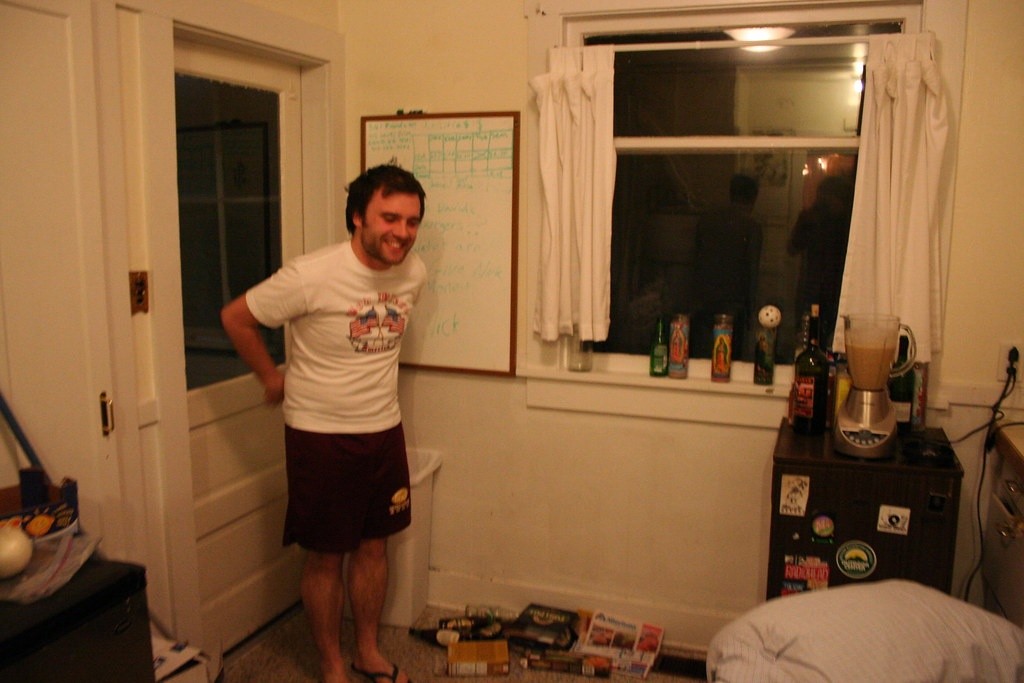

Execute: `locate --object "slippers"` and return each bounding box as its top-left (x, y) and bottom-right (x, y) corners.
top-left (351, 656), bottom-right (412, 683)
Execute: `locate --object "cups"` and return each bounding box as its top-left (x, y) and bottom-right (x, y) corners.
top-left (570, 327), bottom-right (593, 372)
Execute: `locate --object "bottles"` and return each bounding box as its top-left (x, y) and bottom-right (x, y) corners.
top-left (667, 314), bottom-right (689, 379)
top-left (466, 604), bottom-right (520, 623)
top-left (754, 325), bottom-right (777, 384)
top-left (439, 617), bottom-right (500, 632)
top-left (461, 621), bottom-right (503, 639)
top-left (409, 628), bottom-right (465, 649)
top-left (521, 655), bottom-right (613, 679)
top-left (711, 314), bottom-right (733, 383)
top-left (787, 304), bottom-right (837, 442)
top-left (886, 336), bottom-right (915, 436)
top-left (650, 317), bottom-right (668, 377)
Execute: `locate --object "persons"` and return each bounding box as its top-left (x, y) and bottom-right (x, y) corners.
top-left (221, 163), bottom-right (428, 683)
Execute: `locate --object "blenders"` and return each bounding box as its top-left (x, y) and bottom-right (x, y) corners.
top-left (833, 312), bottom-right (916, 458)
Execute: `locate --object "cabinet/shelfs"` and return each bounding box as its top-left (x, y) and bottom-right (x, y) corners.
top-left (767, 417), bottom-right (964, 607)
top-left (983, 429), bottom-right (1024, 627)
top-left (0, 559), bottom-right (156, 683)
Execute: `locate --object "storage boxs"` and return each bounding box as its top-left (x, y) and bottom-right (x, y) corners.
top-left (152, 637), bottom-right (211, 683)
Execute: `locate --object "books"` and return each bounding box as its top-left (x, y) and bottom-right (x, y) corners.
top-left (448, 640), bottom-right (510, 677)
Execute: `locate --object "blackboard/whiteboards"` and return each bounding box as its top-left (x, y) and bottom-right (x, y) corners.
top-left (360, 110), bottom-right (521, 377)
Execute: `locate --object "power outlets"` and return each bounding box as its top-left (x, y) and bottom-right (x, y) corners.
top-left (996, 340), bottom-right (1023, 382)
